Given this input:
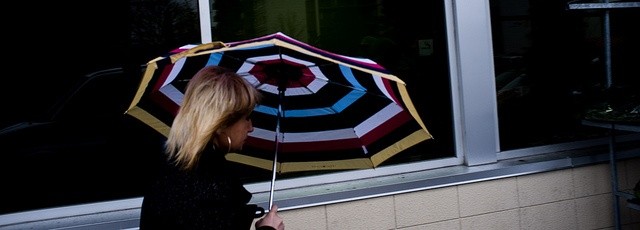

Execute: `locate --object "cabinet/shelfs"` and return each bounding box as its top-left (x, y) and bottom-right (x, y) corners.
top-left (565, 0), bottom-right (640, 230)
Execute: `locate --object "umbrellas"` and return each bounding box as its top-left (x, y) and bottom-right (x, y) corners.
top-left (123, 32), bottom-right (434, 213)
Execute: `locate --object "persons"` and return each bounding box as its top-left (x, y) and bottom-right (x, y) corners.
top-left (139, 66), bottom-right (286, 229)
